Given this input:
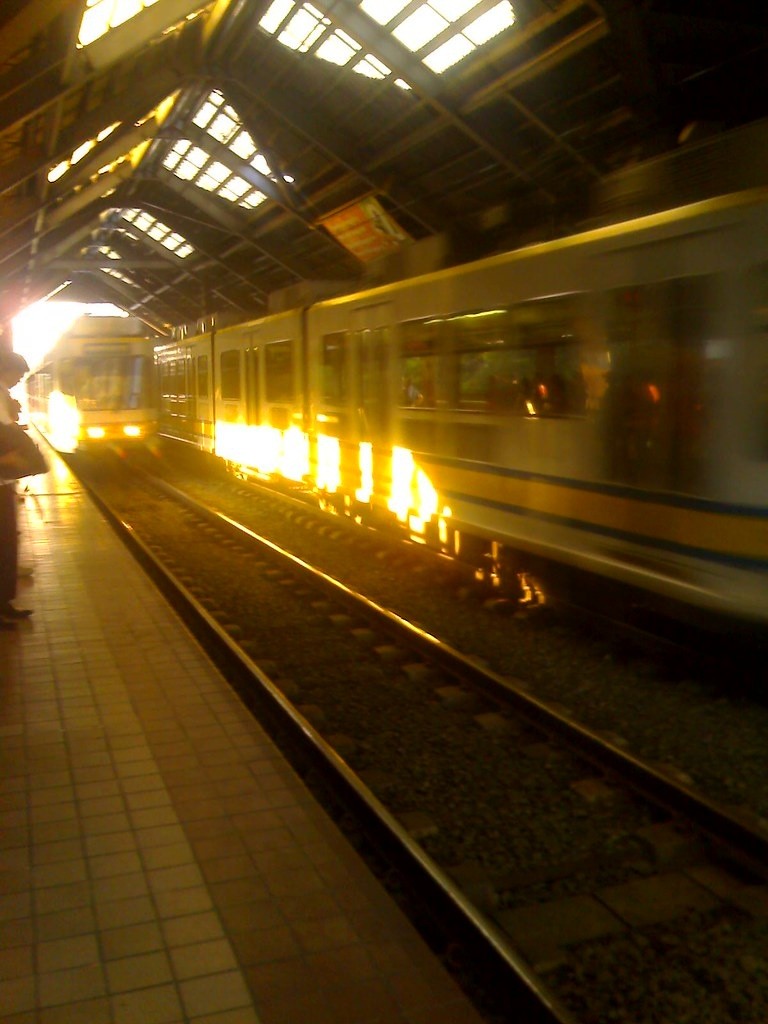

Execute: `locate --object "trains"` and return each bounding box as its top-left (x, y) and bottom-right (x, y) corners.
top-left (156, 184), bottom-right (768, 634)
top-left (24, 315), bottom-right (159, 468)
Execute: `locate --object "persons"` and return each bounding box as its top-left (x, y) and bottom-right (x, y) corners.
top-left (0, 354), bottom-right (50, 629)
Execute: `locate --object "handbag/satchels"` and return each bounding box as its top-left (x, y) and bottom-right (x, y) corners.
top-left (0, 424), bottom-right (48, 481)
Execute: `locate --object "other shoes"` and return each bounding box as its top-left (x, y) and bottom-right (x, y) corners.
top-left (16, 567), bottom-right (33, 576)
top-left (0, 603), bottom-right (32, 617)
